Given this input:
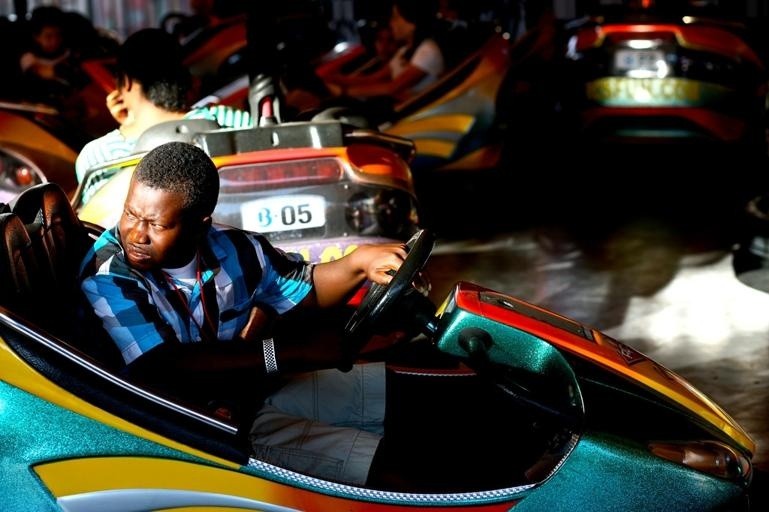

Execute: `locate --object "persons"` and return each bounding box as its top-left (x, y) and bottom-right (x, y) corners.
top-left (80, 141), bottom-right (540, 490)
top-left (0, 0), bottom-right (442, 200)
